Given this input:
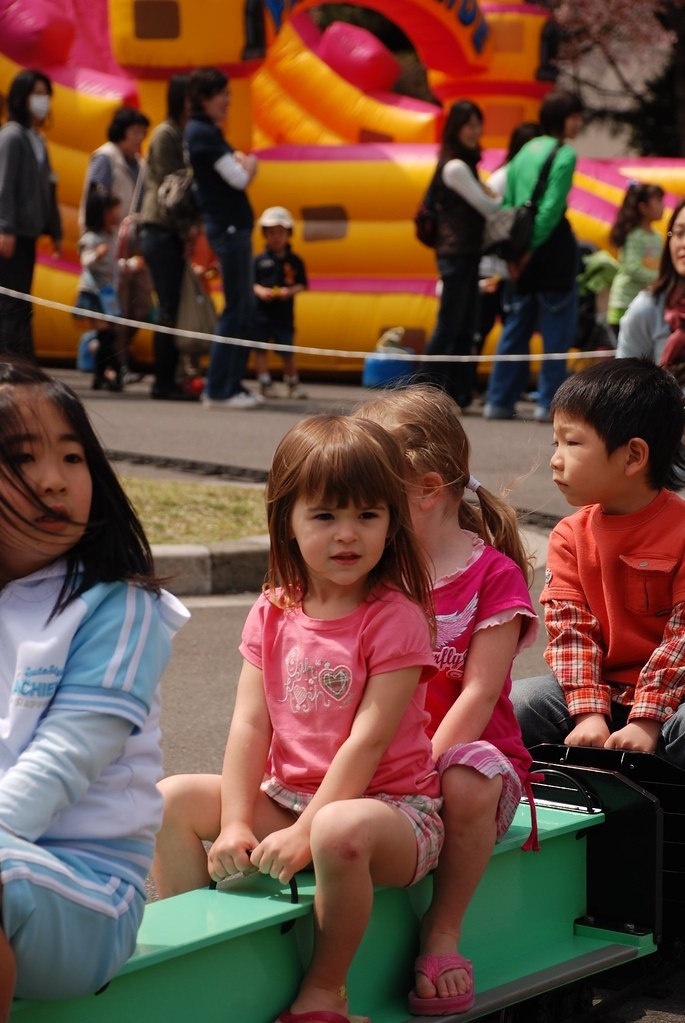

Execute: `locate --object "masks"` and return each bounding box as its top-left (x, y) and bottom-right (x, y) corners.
top-left (30, 94), bottom-right (50, 120)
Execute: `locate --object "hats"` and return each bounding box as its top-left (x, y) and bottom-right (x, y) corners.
top-left (258, 206), bottom-right (294, 229)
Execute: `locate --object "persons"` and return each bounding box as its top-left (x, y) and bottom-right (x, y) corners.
top-left (472, 92), bottom-right (580, 424)
top-left (144, 412), bottom-right (443, 1023)
top-left (182, 65), bottom-right (269, 409)
top-left (0, 68), bottom-right (64, 367)
top-left (71, 107), bottom-right (151, 396)
top-left (135, 72), bottom-right (203, 407)
top-left (606, 180), bottom-right (685, 393)
top-left (413, 99), bottom-right (504, 413)
top-left (0, 356), bottom-right (192, 1023)
top-left (250, 205), bottom-right (311, 401)
top-left (512, 358), bottom-right (685, 942)
top-left (348, 383), bottom-right (543, 1015)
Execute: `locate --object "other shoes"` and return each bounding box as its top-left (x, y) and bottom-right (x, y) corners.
top-left (115, 349), bottom-right (139, 385)
top-left (149, 384), bottom-right (199, 401)
top-left (261, 381), bottom-right (283, 398)
top-left (289, 383), bottom-right (306, 398)
top-left (533, 406), bottom-right (551, 422)
top-left (202, 389), bottom-right (266, 409)
top-left (483, 404), bottom-right (514, 419)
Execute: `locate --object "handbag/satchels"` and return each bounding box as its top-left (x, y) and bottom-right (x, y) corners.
top-left (479, 208), bottom-right (534, 262)
top-left (112, 216), bottom-right (141, 261)
top-left (174, 264), bottom-right (219, 354)
top-left (157, 169), bottom-right (202, 219)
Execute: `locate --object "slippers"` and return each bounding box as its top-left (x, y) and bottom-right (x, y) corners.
top-left (407, 954), bottom-right (475, 1014)
top-left (277, 1010), bottom-right (369, 1023)
